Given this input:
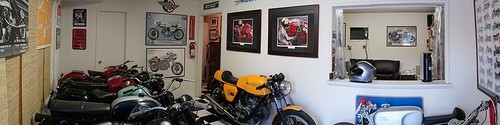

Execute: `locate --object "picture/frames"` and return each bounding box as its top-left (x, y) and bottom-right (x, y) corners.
top-left (211, 17), bottom-right (218, 27)
top-left (386, 25), bottom-right (417, 47)
top-left (73, 9), bottom-right (87, 27)
top-left (146, 47), bottom-right (185, 77)
top-left (227, 9), bottom-right (262, 53)
top-left (209, 29), bottom-right (217, 40)
top-left (145, 12), bottom-right (188, 46)
top-left (268, 4), bottom-right (319, 58)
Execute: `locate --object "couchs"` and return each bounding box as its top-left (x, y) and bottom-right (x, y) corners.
top-left (349, 58), bottom-right (401, 80)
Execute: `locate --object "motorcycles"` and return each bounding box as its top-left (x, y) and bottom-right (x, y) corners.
top-left (148, 21), bottom-right (185, 41)
top-left (148, 53), bottom-right (183, 75)
top-left (35, 61), bottom-right (229, 125)
top-left (201, 69), bottom-right (318, 125)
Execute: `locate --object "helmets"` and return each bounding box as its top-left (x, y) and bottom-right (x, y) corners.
top-left (238, 20), bottom-right (244, 29)
top-left (283, 19), bottom-right (290, 29)
top-left (349, 61), bottom-right (377, 83)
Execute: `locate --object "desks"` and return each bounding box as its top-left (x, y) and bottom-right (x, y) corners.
top-left (399, 75), bottom-right (415, 80)
